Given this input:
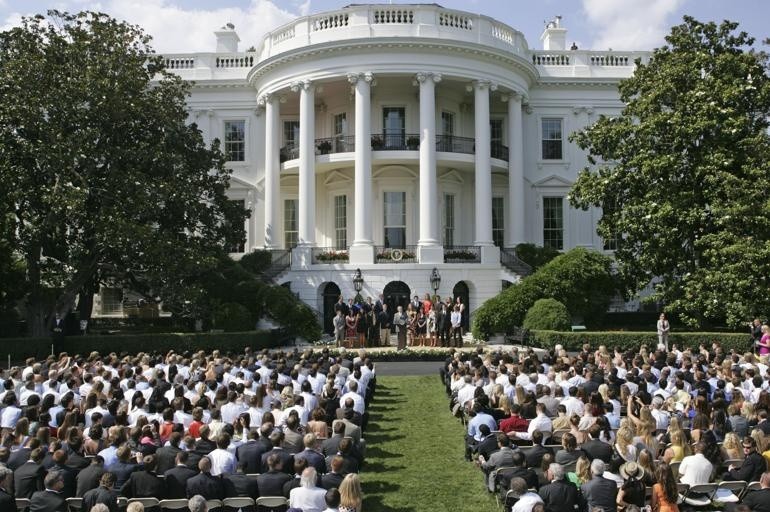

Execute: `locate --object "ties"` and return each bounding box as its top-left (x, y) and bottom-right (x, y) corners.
top-left (662, 321), bottom-right (665, 329)
top-left (444, 312), bottom-right (445, 316)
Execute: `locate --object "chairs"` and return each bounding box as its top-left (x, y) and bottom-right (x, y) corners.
top-left (11, 495), bottom-right (290, 512)
top-left (483, 406), bottom-right (762, 510)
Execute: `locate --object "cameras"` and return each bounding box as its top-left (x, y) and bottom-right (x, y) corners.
top-left (632, 395), bottom-right (637, 401)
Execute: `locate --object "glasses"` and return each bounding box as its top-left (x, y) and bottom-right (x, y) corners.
top-left (742, 444), bottom-right (751, 449)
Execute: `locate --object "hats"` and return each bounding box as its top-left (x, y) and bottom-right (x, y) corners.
top-left (619, 461), bottom-right (645, 481)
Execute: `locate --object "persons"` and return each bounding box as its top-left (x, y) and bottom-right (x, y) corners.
top-left (1, 344), bottom-right (375, 511)
top-left (657, 312), bottom-right (670, 352)
top-left (332, 293), bottom-right (465, 347)
top-left (750, 318), bottom-right (769, 354)
top-left (441, 341), bottom-right (769, 510)
